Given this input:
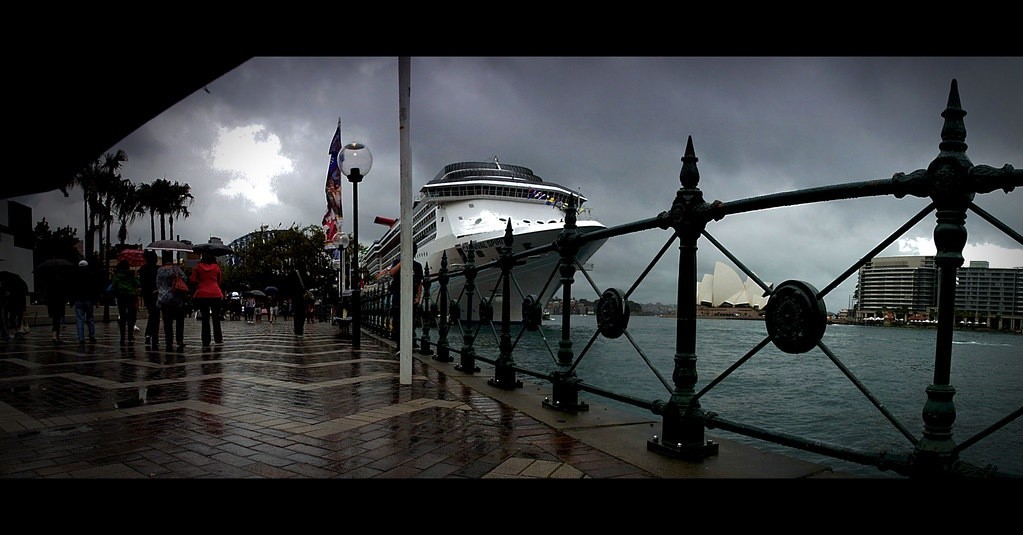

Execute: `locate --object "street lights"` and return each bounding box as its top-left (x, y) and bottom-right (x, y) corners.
top-left (337, 144), bottom-right (373, 344)
top-left (332, 231), bottom-right (350, 317)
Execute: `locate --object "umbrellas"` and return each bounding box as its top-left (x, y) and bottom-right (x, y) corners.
top-left (38, 258), bottom-right (73, 268)
top-left (118, 239), bottom-right (235, 267)
top-left (251, 290), bottom-right (266, 296)
top-left (0, 270), bottom-right (28, 294)
top-left (264, 286), bottom-right (279, 292)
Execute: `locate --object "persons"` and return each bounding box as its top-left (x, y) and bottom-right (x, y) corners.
top-left (47, 260), bottom-right (99, 345)
top-left (287, 261), bottom-right (310, 337)
top-left (390, 241), bottom-right (423, 356)
top-left (0, 281), bottom-right (25, 342)
top-left (112, 252), bottom-right (224, 352)
top-left (223, 293), bottom-right (322, 324)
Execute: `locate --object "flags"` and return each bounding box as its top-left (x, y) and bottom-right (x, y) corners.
top-left (322, 127), bottom-right (342, 243)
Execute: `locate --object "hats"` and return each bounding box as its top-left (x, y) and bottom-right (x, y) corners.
top-left (78, 260), bottom-right (88, 267)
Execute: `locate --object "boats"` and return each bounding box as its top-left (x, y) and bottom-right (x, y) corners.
top-left (543, 312), bottom-right (550, 319)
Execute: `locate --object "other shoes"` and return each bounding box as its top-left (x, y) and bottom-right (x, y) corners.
top-left (152, 339), bottom-right (159, 344)
top-left (89, 337), bottom-right (97, 343)
top-left (248, 321), bottom-right (250, 323)
top-left (251, 321), bottom-right (253, 323)
top-left (395, 349), bottom-right (400, 355)
top-left (145, 341), bottom-right (151, 345)
top-left (214, 342), bottom-right (224, 346)
top-left (177, 342), bottom-right (185, 347)
top-left (202, 342), bottom-right (210, 347)
top-left (270, 321), bottom-right (272, 323)
top-left (52, 334), bottom-right (62, 345)
top-left (128, 336), bottom-right (135, 341)
top-left (79, 339), bottom-right (86, 345)
top-left (120, 340), bottom-right (124, 344)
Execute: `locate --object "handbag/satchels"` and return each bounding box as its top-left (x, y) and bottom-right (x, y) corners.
top-left (172, 265), bottom-right (189, 293)
top-left (304, 290), bottom-right (315, 302)
top-left (17, 316), bottom-right (30, 334)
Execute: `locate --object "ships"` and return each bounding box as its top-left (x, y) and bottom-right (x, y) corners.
top-left (359, 156), bottom-right (610, 323)
